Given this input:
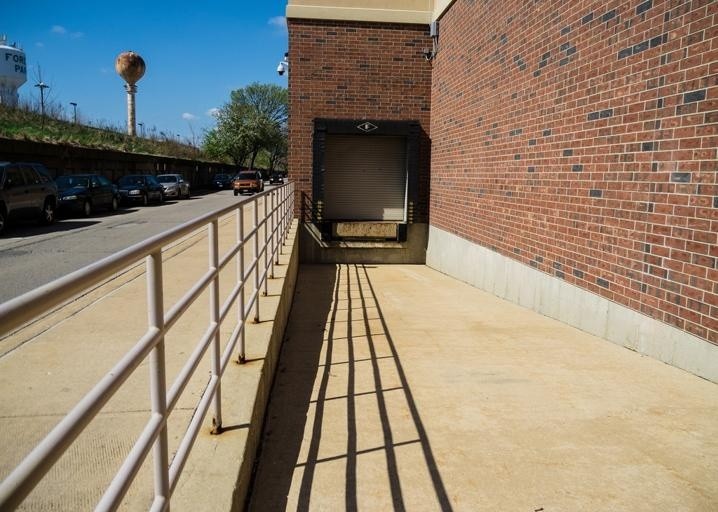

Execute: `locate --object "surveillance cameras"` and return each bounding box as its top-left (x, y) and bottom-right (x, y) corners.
top-left (277, 64), bottom-right (285, 75)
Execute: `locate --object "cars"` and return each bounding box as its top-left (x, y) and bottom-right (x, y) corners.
top-left (212, 172), bottom-right (284, 188)
top-left (0, 160), bottom-right (191, 235)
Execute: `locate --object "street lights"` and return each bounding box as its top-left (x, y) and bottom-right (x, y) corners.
top-left (69, 101), bottom-right (78, 124)
top-left (137, 122), bottom-right (143, 137)
top-left (176, 133), bottom-right (180, 144)
top-left (33, 81), bottom-right (49, 116)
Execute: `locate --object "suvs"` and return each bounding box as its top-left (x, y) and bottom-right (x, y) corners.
top-left (231, 170), bottom-right (264, 195)
